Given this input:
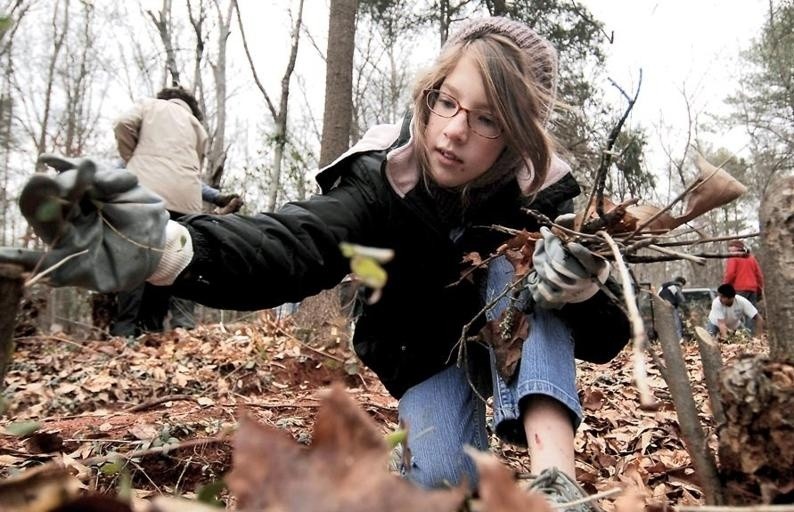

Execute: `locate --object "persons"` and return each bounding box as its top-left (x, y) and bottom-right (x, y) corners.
top-left (705, 283), bottom-right (764, 341)
top-left (721, 239), bottom-right (764, 338)
top-left (109, 82), bottom-right (210, 334)
top-left (655, 275), bottom-right (692, 345)
top-left (17, 15), bottom-right (634, 512)
top-left (200, 182), bottom-right (244, 214)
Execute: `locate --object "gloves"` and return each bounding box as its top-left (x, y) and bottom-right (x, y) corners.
top-left (1, 151), bottom-right (195, 295)
top-left (526, 226), bottom-right (610, 311)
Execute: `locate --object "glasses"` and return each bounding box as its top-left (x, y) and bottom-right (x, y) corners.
top-left (422, 87), bottom-right (507, 140)
